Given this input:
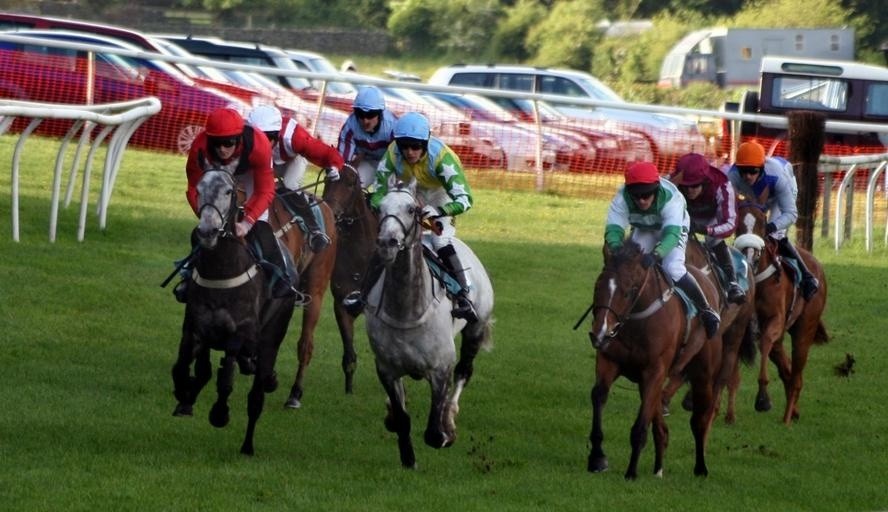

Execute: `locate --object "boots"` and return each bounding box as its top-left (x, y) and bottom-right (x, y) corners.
top-left (438, 248), bottom-right (479, 324)
top-left (256, 224), bottom-right (296, 297)
top-left (173, 225), bottom-right (198, 296)
top-left (283, 188), bottom-right (330, 248)
top-left (778, 236), bottom-right (821, 301)
top-left (344, 249), bottom-right (384, 318)
top-left (710, 238), bottom-right (746, 300)
top-left (672, 271), bottom-right (720, 340)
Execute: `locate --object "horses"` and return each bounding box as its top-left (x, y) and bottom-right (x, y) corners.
top-left (734, 186), bottom-right (833, 430)
top-left (322, 142), bottom-right (376, 403)
top-left (270, 184), bottom-right (336, 409)
top-left (169, 147), bottom-right (297, 455)
top-left (585, 237), bottom-right (756, 484)
top-left (363, 172), bottom-right (493, 468)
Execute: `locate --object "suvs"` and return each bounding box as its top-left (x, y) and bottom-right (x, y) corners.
top-left (428, 61), bottom-right (697, 133)
top-left (721, 55), bottom-right (888, 190)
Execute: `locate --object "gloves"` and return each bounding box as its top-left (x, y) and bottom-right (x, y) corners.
top-left (641, 251), bottom-right (661, 270)
top-left (767, 223), bottom-right (776, 236)
top-left (422, 205), bottom-right (440, 221)
top-left (324, 166), bottom-right (340, 181)
top-left (234, 218), bottom-right (250, 237)
top-left (693, 225), bottom-right (707, 235)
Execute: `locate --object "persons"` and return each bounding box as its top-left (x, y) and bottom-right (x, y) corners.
top-left (663, 153), bottom-right (746, 302)
top-left (246, 103), bottom-right (344, 246)
top-left (726, 141), bottom-right (819, 303)
top-left (338, 86), bottom-right (398, 190)
top-left (343, 111), bottom-right (478, 324)
top-left (176, 109), bottom-right (295, 302)
top-left (604, 161), bottom-right (721, 340)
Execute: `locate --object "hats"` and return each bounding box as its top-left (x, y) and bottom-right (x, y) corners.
top-left (735, 141), bottom-right (766, 166)
top-left (204, 108), bottom-right (244, 137)
top-left (623, 162), bottom-right (661, 194)
top-left (350, 85), bottom-right (386, 111)
top-left (392, 112), bottom-right (432, 141)
top-left (248, 105), bottom-right (283, 131)
top-left (674, 153), bottom-right (714, 187)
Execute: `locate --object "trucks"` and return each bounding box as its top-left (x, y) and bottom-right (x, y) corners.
top-left (657, 26), bottom-right (855, 93)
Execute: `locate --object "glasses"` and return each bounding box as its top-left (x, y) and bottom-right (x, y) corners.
top-left (210, 138), bottom-right (237, 148)
top-left (397, 143), bottom-right (422, 152)
top-left (266, 134), bottom-right (275, 140)
top-left (680, 183), bottom-right (704, 190)
top-left (738, 167), bottom-right (760, 176)
top-left (356, 110), bottom-right (379, 119)
top-left (631, 194), bottom-right (654, 200)
